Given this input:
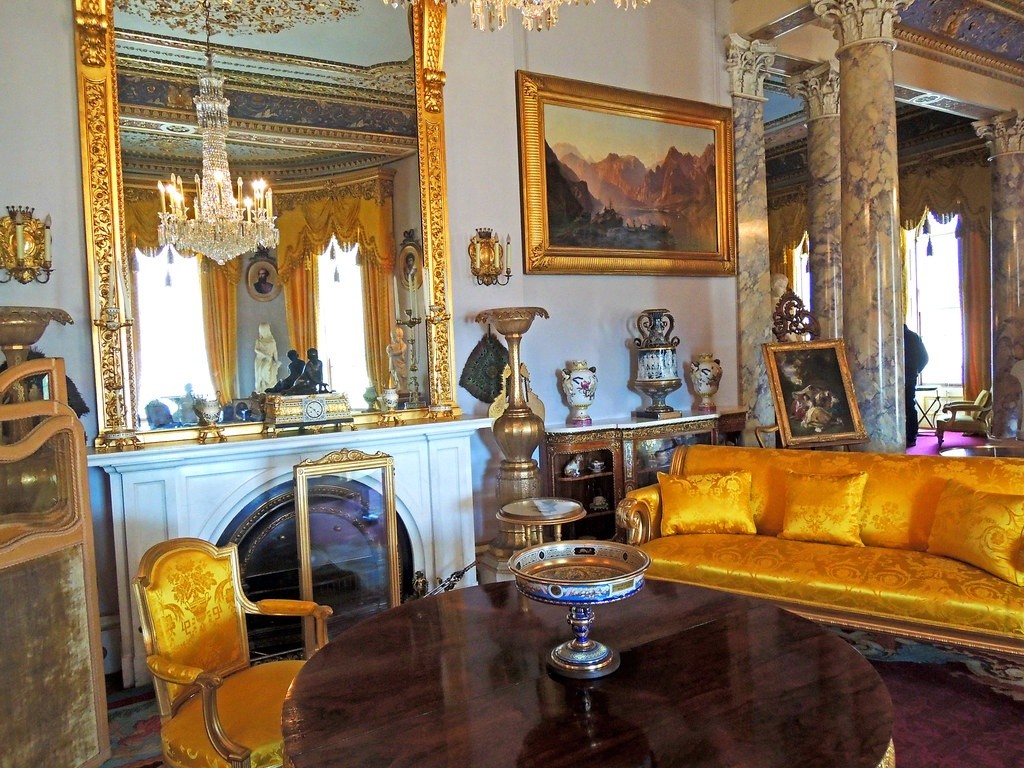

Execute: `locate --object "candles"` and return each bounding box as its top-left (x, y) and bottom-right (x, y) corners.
top-left (393, 274), bottom-right (401, 320)
top-left (408, 273), bottom-right (416, 318)
top-left (413, 273), bottom-right (420, 318)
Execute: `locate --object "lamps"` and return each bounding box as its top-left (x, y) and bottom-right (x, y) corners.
top-left (0, 205), bottom-right (57, 285)
top-left (382, 0), bottom-right (652, 34)
top-left (468, 227), bottom-right (514, 287)
top-left (157, 0), bottom-right (280, 265)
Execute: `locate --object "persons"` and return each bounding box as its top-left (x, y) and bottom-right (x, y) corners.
top-left (385, 326), bottom-right (421, 395)
top-left (265, 351), bottom-right (306, 394)
top-left (903, 323), bottom-right (929, 449)
top-left (254, 324), bottom-right (278, 394)
top-left (279, 348), bottom-right (330, 394)
top-left (254, 269), bottom-right (273, 293)
top-left (404, 254), bottom-right (417, 281)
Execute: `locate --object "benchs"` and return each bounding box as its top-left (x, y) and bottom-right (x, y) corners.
top-left (614, 444), bottom-right (1024, 663)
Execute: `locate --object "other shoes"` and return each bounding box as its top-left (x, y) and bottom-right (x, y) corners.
top-left (906, 439), bottom-right (916, 447)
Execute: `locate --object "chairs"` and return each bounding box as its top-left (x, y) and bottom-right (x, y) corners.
top-left (935, 389), bottom-right (992, 450)
top-left (131, 536), bottom-right (333, 768)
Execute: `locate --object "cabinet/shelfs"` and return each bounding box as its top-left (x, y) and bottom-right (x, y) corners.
top-left (538, 405), bottom-right (749, 542)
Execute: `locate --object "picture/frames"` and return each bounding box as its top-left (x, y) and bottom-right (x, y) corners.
top-left (398, 243), bottom-right (423, 292)
top-left (760, 338), bottom-right (872, 449)
top-left (245, 259), bottom-right (282, 303)
top-left (514, 69), bottom-right (739, 278)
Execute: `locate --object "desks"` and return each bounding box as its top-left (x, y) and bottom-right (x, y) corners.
top-left (914, 386), bottom-right (941, 436)
top-left (280, 578), bottom-right (897, 768)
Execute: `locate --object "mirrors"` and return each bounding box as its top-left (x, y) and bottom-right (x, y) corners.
top-left (70, 1), bottom-right (463, 450)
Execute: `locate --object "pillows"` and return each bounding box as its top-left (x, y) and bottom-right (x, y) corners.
top-left (657, 468), bottom-right (757, 537)
top-left (925, 477), bottom-right (1024, 587)
top-left (776, 465), bottom-right (869, 548)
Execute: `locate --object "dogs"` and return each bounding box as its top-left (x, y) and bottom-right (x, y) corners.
top-left (563, 453), bottom-right (584, 478)
